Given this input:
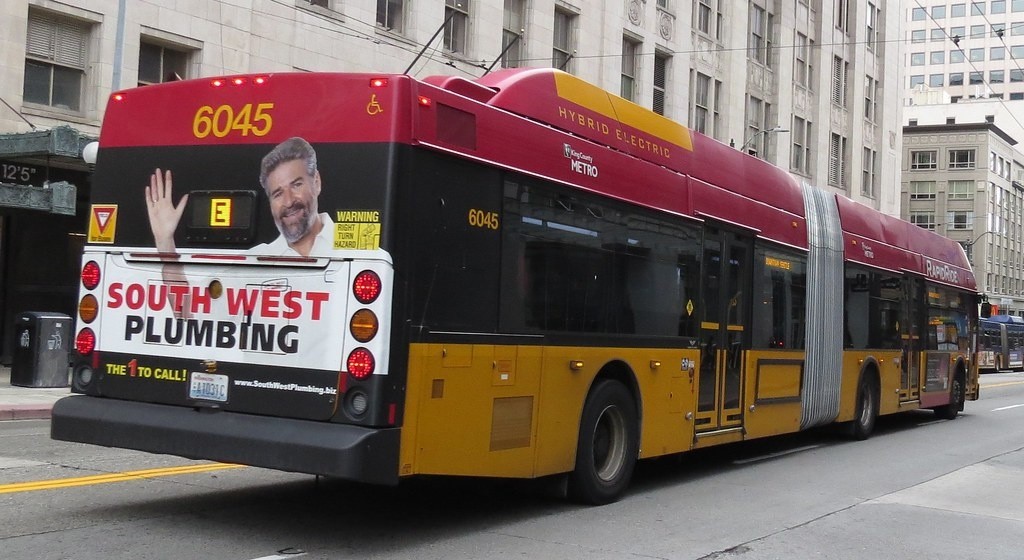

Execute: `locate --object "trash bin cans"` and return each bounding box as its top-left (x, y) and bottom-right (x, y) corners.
top-left (9, 310), bottom-right (74, 390)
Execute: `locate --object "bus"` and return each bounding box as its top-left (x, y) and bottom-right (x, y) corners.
top-left (976, 315), bottom-right (1024, 373)
top-left (47, 66), bottom-right (992, 508)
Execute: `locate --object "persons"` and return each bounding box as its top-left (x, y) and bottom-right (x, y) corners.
top-left (143, 137), bottom-right (394, 377)
